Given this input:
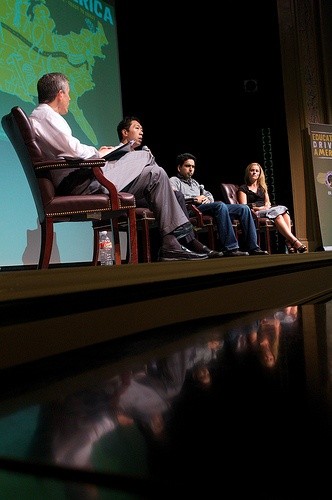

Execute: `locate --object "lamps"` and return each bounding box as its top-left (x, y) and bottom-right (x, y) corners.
top-left (261, 127), bottom-right (278, 243)
top-left (243, 79), bottom-right (258, 92)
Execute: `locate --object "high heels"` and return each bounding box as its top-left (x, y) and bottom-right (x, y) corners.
top-left (284, 240), bottom-right (297, 253)
top-left (293, 240), bottom-right (308, 253)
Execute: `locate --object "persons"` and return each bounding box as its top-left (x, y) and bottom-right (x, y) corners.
top-left (250, 303), bottom-right (298, 369)
top-left (116, 352), bottom-right (183, 434)
top-left (29, 73), bottom-right (208, 262)
top-left (237, 163), bottom-right (308, 254)
top-left (98, 117), bottom-right (223, 259)
top-left (51, 408), bottom-right (126, 477)
top-left (184, 339), bottom-right (224, 386)
top-left (169, 153), bottom-right (268, 256)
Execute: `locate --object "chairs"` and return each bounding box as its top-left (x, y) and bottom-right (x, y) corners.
top-left (186, 203), bottom-right (239, 251)
top-left (220, 183), bottom-right (294, 255)
top-left (1, 106), bottom-right (139, 270)
top-left (91, 207), bottom-right (157, 266)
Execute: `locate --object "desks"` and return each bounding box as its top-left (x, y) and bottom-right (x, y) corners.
top-left (0, 302), bottom-right (332, 500)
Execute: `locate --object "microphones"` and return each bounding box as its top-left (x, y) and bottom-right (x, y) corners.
top-left (199, 185), bottom-right (205, 196)
top-left (142, 146), bottom-right (148, 150)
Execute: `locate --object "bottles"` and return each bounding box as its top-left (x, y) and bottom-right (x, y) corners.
top-left (99, 231), bottom-right (113, 265)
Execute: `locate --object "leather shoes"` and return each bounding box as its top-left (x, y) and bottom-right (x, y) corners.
top-left (201, 245), bottom-right (223, 257)
top-left (162, 245), bottom-right (210, 261)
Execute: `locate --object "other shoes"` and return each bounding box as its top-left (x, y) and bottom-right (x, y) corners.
top-left (226, 249), bottom-right (249, 256)
top-left (252, 247), bottom-right (269, 256)
